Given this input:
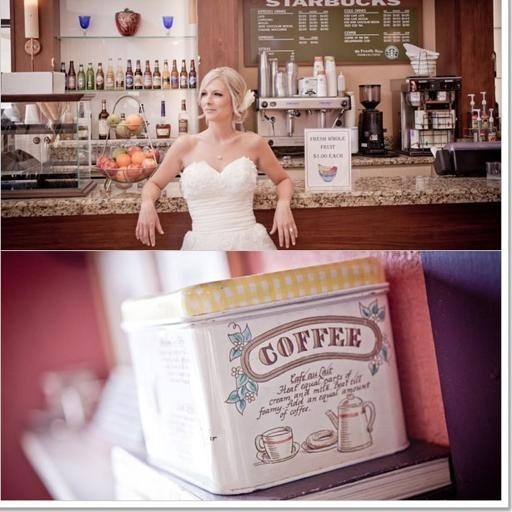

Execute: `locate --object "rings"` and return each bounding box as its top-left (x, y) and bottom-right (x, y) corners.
top-left (290, 229), bottom-right (293, 231)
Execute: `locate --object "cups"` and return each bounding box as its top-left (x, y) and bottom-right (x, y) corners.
top-left (316, 72), bottom-right (326, 97)
top-left (313, 57), bottom-right (323, 76)
top-left (324, 57), bottom-right (337, 97)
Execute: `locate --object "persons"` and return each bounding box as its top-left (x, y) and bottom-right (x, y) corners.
top-left (135, 66), bottom-right (299, 250)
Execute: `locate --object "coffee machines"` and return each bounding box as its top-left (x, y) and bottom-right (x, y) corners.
top-left (358, 84), bottom-right (382, 153)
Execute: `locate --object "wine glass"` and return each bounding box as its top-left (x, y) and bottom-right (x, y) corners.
top-left (79, 15), bottom-right (91, 36)
top-left (163, 15), bottom-right (174, 34)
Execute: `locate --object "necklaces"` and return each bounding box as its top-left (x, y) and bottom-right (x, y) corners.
top-left (200, 133), bottom-right (233, 159)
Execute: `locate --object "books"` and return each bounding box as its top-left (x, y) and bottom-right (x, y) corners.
top-left (109, 448), bottom-right (453, 501)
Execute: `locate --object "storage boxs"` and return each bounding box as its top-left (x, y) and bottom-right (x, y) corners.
top-left (122, 256), bottom-right (408, 494)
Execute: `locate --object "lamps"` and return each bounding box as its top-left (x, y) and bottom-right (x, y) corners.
top-left (23, 0), bottom-right (40, 72)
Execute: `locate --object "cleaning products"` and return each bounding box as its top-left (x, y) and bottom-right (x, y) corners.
top-left (480, 91), bottom-right (498, 142)
top-left (469, 91), bottom-right (486, 144)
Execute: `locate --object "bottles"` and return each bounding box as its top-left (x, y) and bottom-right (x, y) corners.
top-left (269, 58), bottom-right (277, 97)
top-left (96, 63), bottom-right (104, 90)
top-left (153, 60), bottom-right (161, 88)
top-left (258, 51), bottom-right (271, 97)
top-left (60, 63), bottom-right (67, 89)
top-left (106, 58), bottom-right (114, 90)
top-left (116, 58), bottom-right (124, 87)
top-left (189, 59), bottom-right (196, 88)
top-left (324, 56), bottom-right (335, 72)
top-left (77, 102), bottom-right (87, 139)
top-left (87, 62), bottom-right (94, 90)
top-left (143, 60), bottom-right (152, 88)
top-left (317, 70), bottom-right (327, 96)
top-left (162, 59), bottom-right (170, 89)
top-left (314, 56), bottom-right (323, 77)
top-left (171, 59), bottom-right (178, 89)
top-left (99, 99), bottom-right (110, 139)
top-left (69, 61), bottom-right (76, 90)
top-left (134, 59), bottom-right (142, 89)
top-left (77, 64), bottom-right (85, 90)
top-left (179, 99), bottom-right (188, 136)
top-left (125, 59), bottom-right (134, 88)
top-left (337, 72), bottom-right (346, 91)
top-left (157, 100), bottom-right (171, 138)
top-left (64, 103), bottom-right (74, 140)
top-left (180, 59), bottom-right (188, 88)
top-left (326, 61), bottom-right (337, 97)
top-left (286, 50), bottom-right (297, 97)
top-left (480, 129), bottom-right (486, 141)
top-left (488, 128), bottom-right (496, 143)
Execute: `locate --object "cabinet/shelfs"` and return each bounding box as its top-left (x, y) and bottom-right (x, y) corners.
top-left (1, 94), bottom-right (98, 199)
top-left (58, 0), bottom-right (199, 143)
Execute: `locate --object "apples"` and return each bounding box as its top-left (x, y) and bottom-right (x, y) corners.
top-left (115, 123), bottom-right (129, 137)
top-left (106, 114), bottom-right (119, 127)
top-left (97, 145), bottom-right (161, 184)
